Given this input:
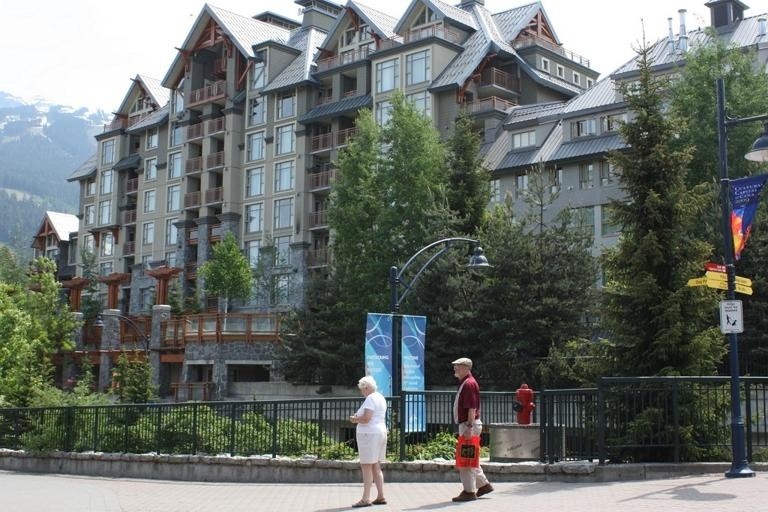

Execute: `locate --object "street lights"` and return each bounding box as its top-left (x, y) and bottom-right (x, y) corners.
top-left (391, 237), bottom-right (495, 427)
top-left (704, 77), bottom-right (768, 477)
top-left (93, 312), bottom-right (150, 402)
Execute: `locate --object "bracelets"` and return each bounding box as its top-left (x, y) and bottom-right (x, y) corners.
top-left (467, 424), bottom-right (473, 428)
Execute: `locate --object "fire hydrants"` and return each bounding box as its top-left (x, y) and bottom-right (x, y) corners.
top-left (511, 385), bottom-right (536, 424)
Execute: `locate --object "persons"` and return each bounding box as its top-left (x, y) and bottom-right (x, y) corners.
top-left (349, 375), bottom-right (389, 507)
top-left (450, 357), bottom-right (494, 501)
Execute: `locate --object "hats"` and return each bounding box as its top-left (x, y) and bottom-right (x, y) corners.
top-left (452, 358), bottom-right (472, 367)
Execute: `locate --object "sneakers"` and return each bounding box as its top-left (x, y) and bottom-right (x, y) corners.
top-left (452, 491), bottom-right (476, 501)
top-left (476, 484), bottom-right (493, 497)
top-left (352, 499), bottom-right (371, 507)
top-left (372, 498), bottom-right (386, 504)
